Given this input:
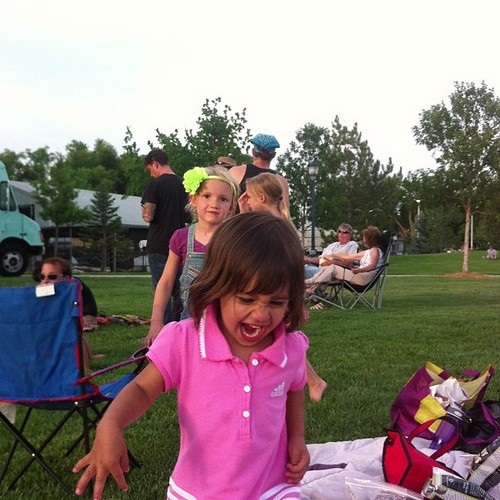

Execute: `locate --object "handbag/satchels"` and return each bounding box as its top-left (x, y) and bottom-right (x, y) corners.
top-left (390, 361), bottom-right (494, 440)
top-left (382, 416), bottom-right (466, 493)
top-left (452, 399), bottom-right (500, 454)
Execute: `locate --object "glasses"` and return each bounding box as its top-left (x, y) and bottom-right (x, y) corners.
top-left (338, 230), bottom-right (349, 234)
top-left (215, 161), bottom-right (227, 165)
top-left (38, 274), bottom-right (63, 280)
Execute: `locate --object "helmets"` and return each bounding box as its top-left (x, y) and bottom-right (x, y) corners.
top-left (251, 134), bottom-right (280, 149)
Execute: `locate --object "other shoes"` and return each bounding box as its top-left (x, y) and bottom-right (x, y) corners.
top-left (309, 302), bottom-right (329, 310)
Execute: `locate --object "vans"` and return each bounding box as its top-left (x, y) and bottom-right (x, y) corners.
top-left (0, 158), bottom-right (46, 277)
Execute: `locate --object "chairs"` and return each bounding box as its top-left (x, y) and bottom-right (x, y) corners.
top-left (0, 280), bottom-right (149, 500)
top-left (304, 231), bottom-right (393, 311)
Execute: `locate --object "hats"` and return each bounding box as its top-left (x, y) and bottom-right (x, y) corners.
top-left (216, 157), bottom-right (236, 167)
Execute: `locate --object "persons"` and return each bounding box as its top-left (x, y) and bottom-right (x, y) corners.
top-left (32, 255), bottom-right (106, 384)
top-left (486, 245), bottom-right (497, 260)
top-left (304, 222), bottom-right (387, 311)
top-left (73, 209), bottom-right (310, 500)
top-left (140, 134), bottom-right (290, 348)
